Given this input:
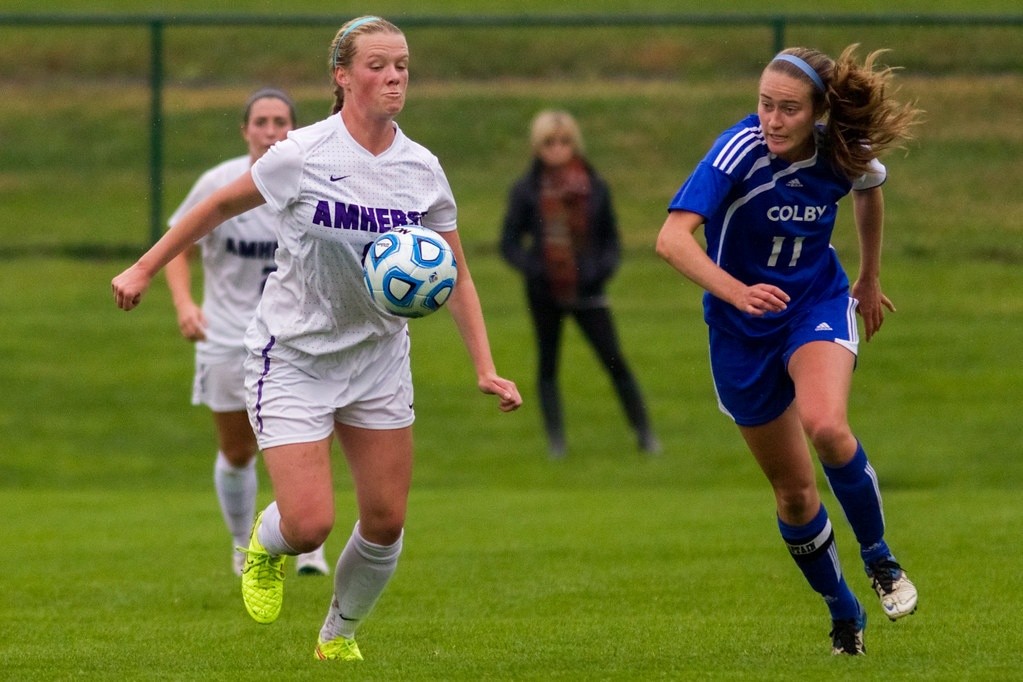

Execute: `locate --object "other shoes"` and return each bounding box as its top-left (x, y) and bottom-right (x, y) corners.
top-left (232, 555), bottom-right (246, 576)
top-left (297, 544), bottom-right (328, 576)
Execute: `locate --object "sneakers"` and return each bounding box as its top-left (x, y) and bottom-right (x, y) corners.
top-left (867, 555), bottom-right (918, 621)
top-left (827, 610), bottom-right (867, 657)
top-left (313, 630), bottom-right (364, 662)
top-left (234, 511), bottom-right (286, 624)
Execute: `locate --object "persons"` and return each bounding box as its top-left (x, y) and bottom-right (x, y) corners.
top-left (164, 90), bottom-right (329, 579)
top-left (110, 16), bottom-right (522, 661)
top-left (498, 109), bottom-right (654, 454)
top-left (652, 38), bottom-right (926, 658)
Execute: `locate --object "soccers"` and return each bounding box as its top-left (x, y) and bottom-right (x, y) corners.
top-left (362, 224), bottom-right (459, 320)
